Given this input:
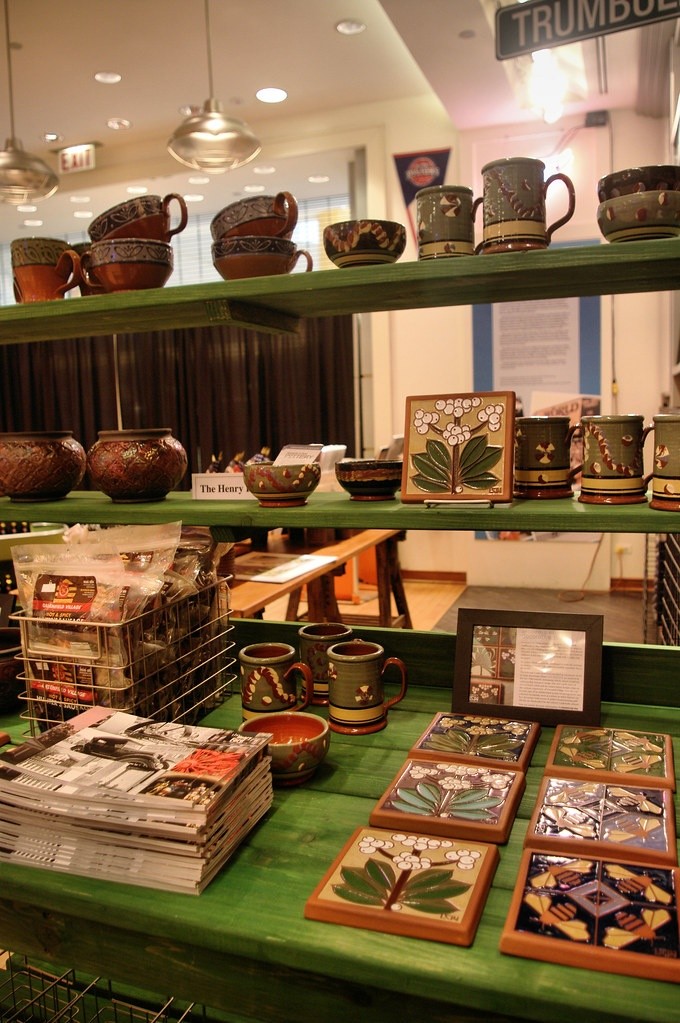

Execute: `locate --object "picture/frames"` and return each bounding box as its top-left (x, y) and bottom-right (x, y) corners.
top-left (304, 710), bottom-right (678, 983)
top-left (449, 609), bottom-right (604, 728)
top-left (401, 391), bottom-right (516, 502)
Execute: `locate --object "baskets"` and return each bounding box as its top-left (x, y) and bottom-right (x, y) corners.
top-left (8, 573), bottom-right (238, 740)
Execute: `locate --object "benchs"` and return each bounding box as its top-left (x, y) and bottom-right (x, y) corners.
top-left (223, 529), bottom-right (414, 630)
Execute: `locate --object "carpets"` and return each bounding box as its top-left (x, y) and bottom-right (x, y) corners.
top-left (431, 585), bottom-right (659, 646)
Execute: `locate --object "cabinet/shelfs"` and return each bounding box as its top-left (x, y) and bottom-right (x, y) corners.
top-left (0, 233), bottom-right (679, 1023)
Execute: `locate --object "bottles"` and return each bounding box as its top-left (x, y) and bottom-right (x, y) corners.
top-left (0, 430), bottom-right (86, 502)
top-left (87, 427), bottom-right (187, 504)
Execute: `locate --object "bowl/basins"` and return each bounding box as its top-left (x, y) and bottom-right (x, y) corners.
top-left (334, 457), bottom-right (403, 501)
top-left (322, 219), bottom-right (405, 270)
top-left (239, 712), bottom-right (331, 787)
top-left (597, 165), bottom-right (680, 243)
top-left (242, 461), bottom-right (321, 508)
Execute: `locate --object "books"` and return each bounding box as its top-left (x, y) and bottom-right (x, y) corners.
top-left (0, 707), bottom-right (273, 894)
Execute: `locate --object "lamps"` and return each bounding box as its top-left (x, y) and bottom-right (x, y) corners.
top-left (165, 1), bottom-right (261, 171)
top-left (0, 1), bottom-right (60, 202)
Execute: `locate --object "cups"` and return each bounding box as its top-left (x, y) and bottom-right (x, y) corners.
top-left (328, 641), bottom-right (407, 734)
top-left (650, 414), bottom-right (680, 510)
top-left (299, 622), bottom-right (362, 707)
top-left (10, 193), bottom-right (188, 304)
top-left (512, 416), bottom-right (585, 499)
top-left (238, 642), bottom-right (314, 720)
top-left (577, 414), bottom-right (655, 505)
top-left (415, 184), bottom-right (484, 262)
top-left (481, 156), bottom-right (576, 256)
top-left (211, 192), bottom-right (313, 279)
